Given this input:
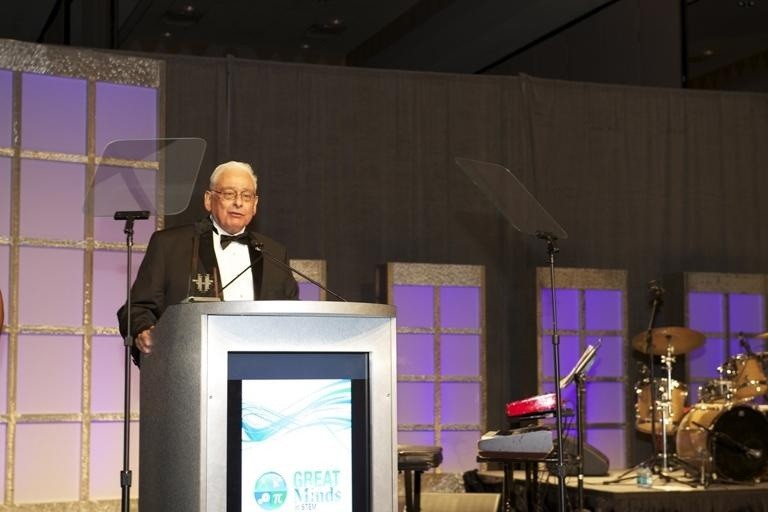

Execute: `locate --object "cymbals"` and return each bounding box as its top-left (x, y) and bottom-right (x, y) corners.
top-left (632, 326), bottom-right (706, 355)
top-left (752, 331), bottom-right (767, 339)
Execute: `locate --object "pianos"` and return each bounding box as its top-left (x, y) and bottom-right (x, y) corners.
top-left (477, 425), bottom-right (554, 454)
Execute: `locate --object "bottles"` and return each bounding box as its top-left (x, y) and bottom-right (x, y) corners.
top-left (637, 461), bottom-right (652, 488)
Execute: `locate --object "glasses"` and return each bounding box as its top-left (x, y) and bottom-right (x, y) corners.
top-left (212, 189), bottom-right (255, 201)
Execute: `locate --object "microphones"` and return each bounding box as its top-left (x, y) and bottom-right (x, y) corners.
top-left (251, 238), bottom-right (349, 302)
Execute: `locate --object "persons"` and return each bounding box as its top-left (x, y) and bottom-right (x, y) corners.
top-left (116, 160), bottom-right (302, 371)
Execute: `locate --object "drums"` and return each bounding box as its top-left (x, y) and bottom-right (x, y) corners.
top-left (717, 351), bottom-right (768, 402)
top-left (633, 376), bottom-right (689, 436)
top-left (676, 397), bottom-right (768, 485)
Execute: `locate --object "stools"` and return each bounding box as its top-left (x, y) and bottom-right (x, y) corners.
top-left (398, 445), bottom-right (444, 512)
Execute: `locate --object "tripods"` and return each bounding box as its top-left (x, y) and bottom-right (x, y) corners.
top-left (605, 280), bottom-right (741, 486)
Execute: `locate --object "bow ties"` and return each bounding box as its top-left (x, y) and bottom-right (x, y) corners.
top-left (212, 226), bottom-right (248, 249)
